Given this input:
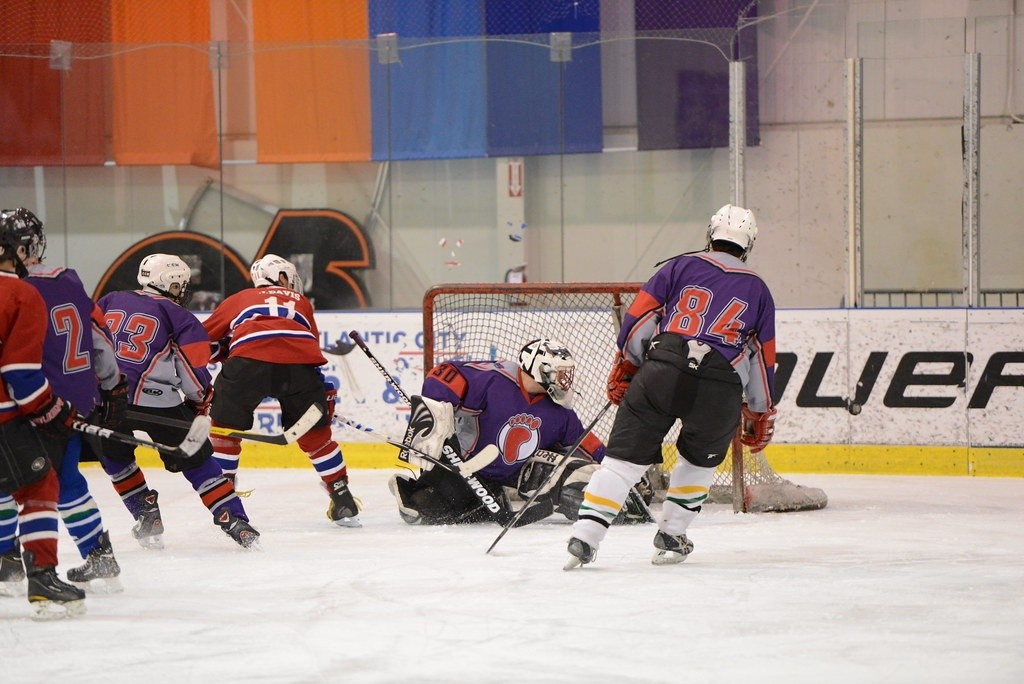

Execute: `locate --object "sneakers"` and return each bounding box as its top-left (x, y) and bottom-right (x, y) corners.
top-left (67, 529), bottom-right (124, 596)
top-left (132, 489), bottom-right (165, 551)
top-left (326, 474), bottom-right (363, 528)
top-left (21, 550), bottom-right (87, 620)
top-left (652, 528), bottom-right (693, 566)
top-left (0, 537), bottom-right (30, 597)
top-left (563, 538), bottom-right (597, 571)
top-left (214, 506), bottom-right (264, 555)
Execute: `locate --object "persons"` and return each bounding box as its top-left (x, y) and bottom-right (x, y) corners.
top-left (17, 229), bottom-right (129, 583)
top-left (200, 254), bottom-right (364, 529)
top-left (0, 207), bottom-right (86, 605)
top-left (568, 204), bottom-right (776, 569)
top-left (392, 338), bottom-right (637, 520)
top-left (92, 253), bottom-right (263, 550)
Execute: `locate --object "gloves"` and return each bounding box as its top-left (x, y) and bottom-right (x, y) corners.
top-left (324, 391), bottom-right (337, 425)
top-left (29, 393), bottom-right (85, 441)
top-left (186, 385), bottom-right (215, 418)
top-left (97, 372), bottom-right (129, 430)
top-left (206, 341), bottom-right (228, 364)
top-left (606, 351), bottom-right (640, 405)
top-left (740, 402), bottom-right (778, 453)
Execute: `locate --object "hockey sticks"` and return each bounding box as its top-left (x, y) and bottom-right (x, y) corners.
top-left (348, 329), bottom-right (555, 529)
top-left (93, 399), bottom-right (326, 447)
top-left (330, 412), bottom-right (501, 478)
top-left (485, 373), bottom-right (633, 554)
top-left (71, 413), bottom-right (212, 459)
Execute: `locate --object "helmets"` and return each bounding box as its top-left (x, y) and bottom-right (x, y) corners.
top-left (708, 203), bottom-right (758, 249)
top-left (137, 254), bottom-right (192, 296)
top-left (0, 206), bottom-right (44, 246)
top-left (518, 338), bottom-right (575, 409)
top-left (250, 255), bottom-right (296, 289)
